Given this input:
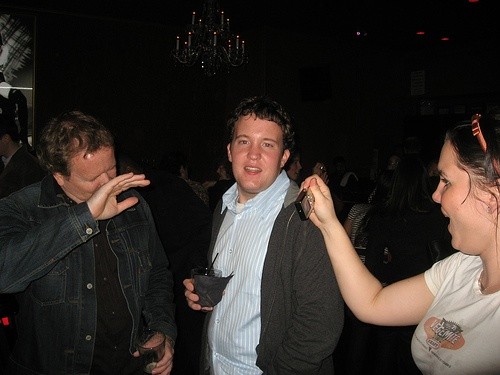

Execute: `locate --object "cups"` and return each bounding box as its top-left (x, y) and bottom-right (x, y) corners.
top-left (137, 328), bottom-right (166, 373)
top-left (191, 269), bottom-right (220, 308)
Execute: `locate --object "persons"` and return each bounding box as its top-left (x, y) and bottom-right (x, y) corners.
top-left (183, 95), bottom-right (344, 375)
top-left (298, 114), bottom-right (500, 374)
top-left (118, 136), bottom-right (500, 375)
top-left (0, 72), bottom-right (45, 200)
top-left (0, 110), bottom-right (176, 375)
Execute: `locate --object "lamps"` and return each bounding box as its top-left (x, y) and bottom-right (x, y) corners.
top-left (169, 0), bottom-right (248, 78)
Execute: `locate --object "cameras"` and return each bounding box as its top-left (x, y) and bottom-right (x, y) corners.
top-left (295, 187), bottom-right (315, 221)
